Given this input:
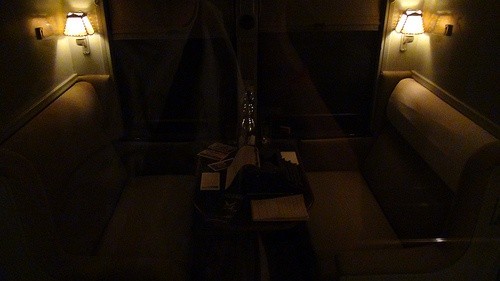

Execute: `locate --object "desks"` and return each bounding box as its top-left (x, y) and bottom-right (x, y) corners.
top-left (193, 183), bottom-right (314, 281)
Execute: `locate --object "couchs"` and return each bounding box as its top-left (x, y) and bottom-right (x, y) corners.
top-left (0, 73), bottom-right (196, 281)
top-left (298, 69), bottom-right (500, 281)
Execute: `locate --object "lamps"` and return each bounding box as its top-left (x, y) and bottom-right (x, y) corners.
top-left (394, 9), bottom-right (425, 52)
top-left (63, 12), bottom-right (94, 56)
